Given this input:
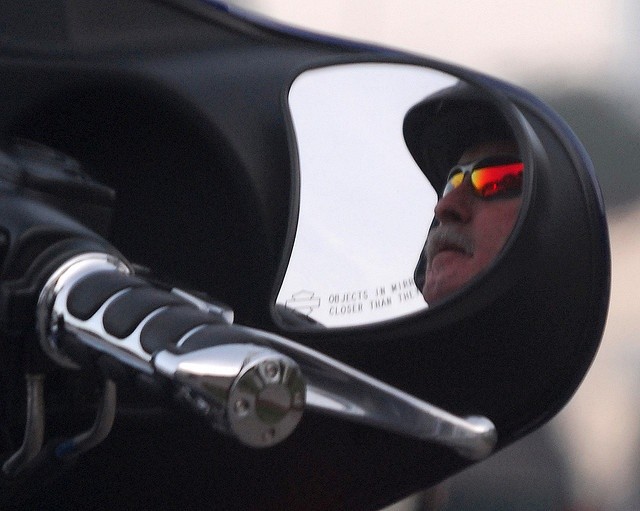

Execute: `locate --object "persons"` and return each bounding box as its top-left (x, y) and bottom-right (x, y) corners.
top-left (402, 79), bottom-right (524, 305)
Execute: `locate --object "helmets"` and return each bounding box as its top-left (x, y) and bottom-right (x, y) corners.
top-left (403, 80), bottom-right (505, 293)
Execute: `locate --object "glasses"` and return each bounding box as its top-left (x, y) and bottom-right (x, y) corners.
top-left (440, 153), bottom-right (524, 201)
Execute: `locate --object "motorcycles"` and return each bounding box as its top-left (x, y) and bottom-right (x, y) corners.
top-left (0, 1), bottom-right (612, 510)
